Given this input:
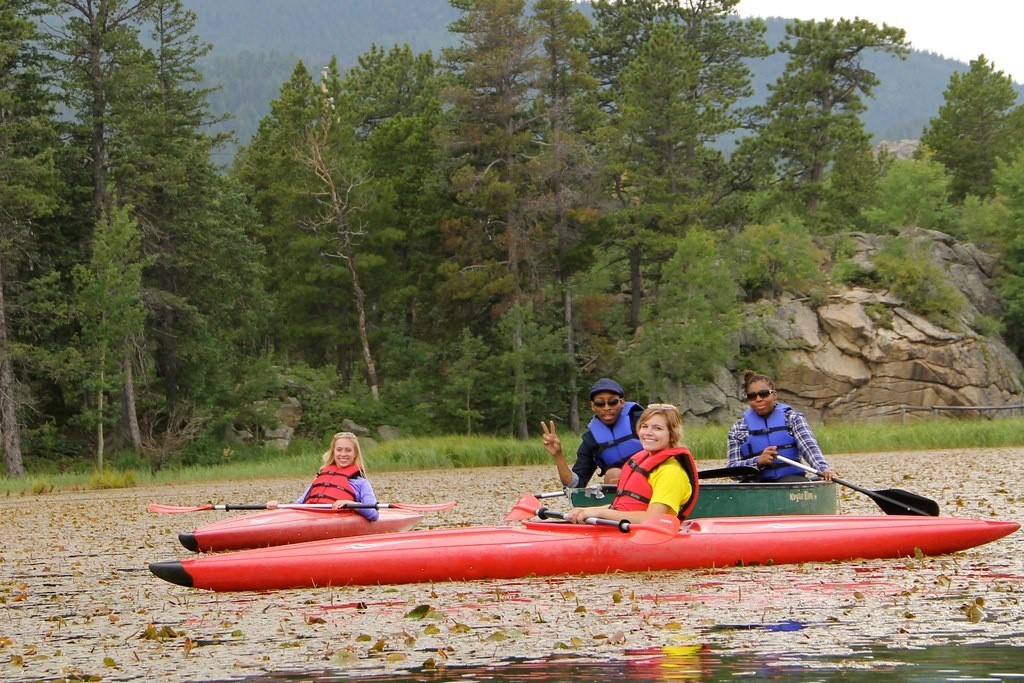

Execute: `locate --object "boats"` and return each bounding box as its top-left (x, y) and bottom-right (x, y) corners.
top-left (148, 515), bottom-right (1021, 595)
top-left (177, 507), bottom-right (427, 554)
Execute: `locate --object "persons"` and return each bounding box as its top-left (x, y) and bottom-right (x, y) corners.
top-left (264, 431), bottom-right (382, 524)
top-left (724, 366), bottom-right (839, 484)
top-left (564, 398), bottom-right (702, 534)
top-left (539, 373), bottom-right (649, 492)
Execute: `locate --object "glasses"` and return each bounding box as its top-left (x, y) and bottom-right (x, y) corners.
top-left (590, 377), bottom-right (624, 402)
top-left (647, 403), bottom-right (676, 411)
top-left (747, 389), bottom-right (776, 401)
top-left (334, 432), bottom-right (356, 439)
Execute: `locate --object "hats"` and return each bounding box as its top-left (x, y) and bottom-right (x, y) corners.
top-left (593, 398), bottom-right (621, 407)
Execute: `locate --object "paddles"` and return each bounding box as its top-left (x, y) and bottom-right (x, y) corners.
top-left (770, 453), bottom-right (940, 517)
top-left (505, 492), bottom-right (680, 543)
top-left (148, 500), bottom-right (458, 513)
top-left (531, 466), bottom-right (760, 498)
